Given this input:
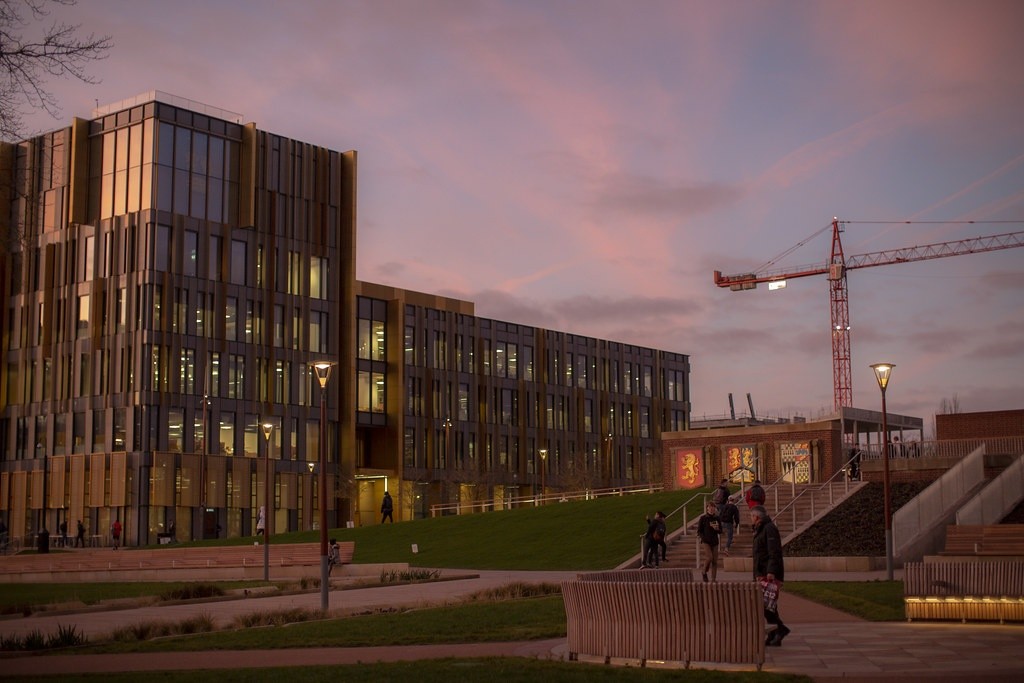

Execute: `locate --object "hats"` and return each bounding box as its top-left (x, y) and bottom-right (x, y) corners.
top-left (330, 539), bottom-right (336, 545)
top-left (662, 514), bottom-right (666, 517)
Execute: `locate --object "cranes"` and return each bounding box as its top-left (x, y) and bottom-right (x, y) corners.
top-left (713, 216), bottom-right (1024, 408)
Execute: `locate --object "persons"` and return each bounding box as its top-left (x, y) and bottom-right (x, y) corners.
top-left (848, 443), bottom-right (860, 481)
top-left (216, 524), bottom-right (222, 539)
top-left (746, 480), bottom-right (765, 510)
top-left (750, 506), bottom-right (790, 647)
top-left (74, 520), bottom-right (85, 548)
top-left (380, 491), bottom-right (393, 523)
top-left (112, 519), bottom-right (122, 550)
top-left (697, 479), bottom-right (740, 583)
top-left (0, 518), bottom-right (7, 544)
top-left (168, 519), bottom-right (175, 543)
top-left (880, 436), bottom-right (920, 458)
top-left (255, 505), bottom-right (265, 536)
top-left (639, 511), bottom-right (669, 570)
top-left (60, 519), bottom-right (70, 548)
top-left (327, 538), bottom-right (340, 577)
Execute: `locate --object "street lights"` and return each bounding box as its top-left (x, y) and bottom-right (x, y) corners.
top-left (256, 421), bottom-right (282, 581)
top-left (604, 433), bottom-right (613, 482)
top-left (871, 362), bottom-right (901, 581)
top-left (539, 449), bottom-right (550, 506)
top-left (443, 418), bottom-right (452, 469)
top-left (306, 359), bottom-right (342, 610)
top-left (200, 393), bottom-right (212, 507)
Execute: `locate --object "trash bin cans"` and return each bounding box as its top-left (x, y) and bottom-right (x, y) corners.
top-left (157, 533), bottom-right (174, 544)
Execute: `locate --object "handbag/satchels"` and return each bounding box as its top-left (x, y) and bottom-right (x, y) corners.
top-left (751, 576), bottom-right (783, 613)
top-left (653, 528), bottom-right (664, 542)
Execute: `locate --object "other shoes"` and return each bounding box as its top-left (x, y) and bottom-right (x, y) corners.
top-left (662, 558), bottom-right (669, 562)
top-left (701, 572), bottom-right (708, 582)
top-left (656, 566), bottom-right (659, 569)
top-left (766, 624), bottom-right (790, 645)
top-left (639, 564), bottom-right (647, 570)
top-left (647, 564), bottom-right (653, 568)
top-left (723, 550), bottom-right (729, 556)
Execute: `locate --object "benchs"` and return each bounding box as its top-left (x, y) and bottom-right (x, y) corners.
top-left (940, 524), bottom-right (1024, 556)
top-left (0, 541), bottom-right (354, 575)
top-left (903, 561), bottom-right (1024, 624)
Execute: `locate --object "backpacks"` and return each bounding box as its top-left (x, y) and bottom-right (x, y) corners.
top-left (750, 486), bottom-right (761, 501)
top-left (713, 488), bottom-right (725, 504)
top-left (256, 512), bottom-right (260, 522)
top-left (719, 505), bottom-right (735, 522)
